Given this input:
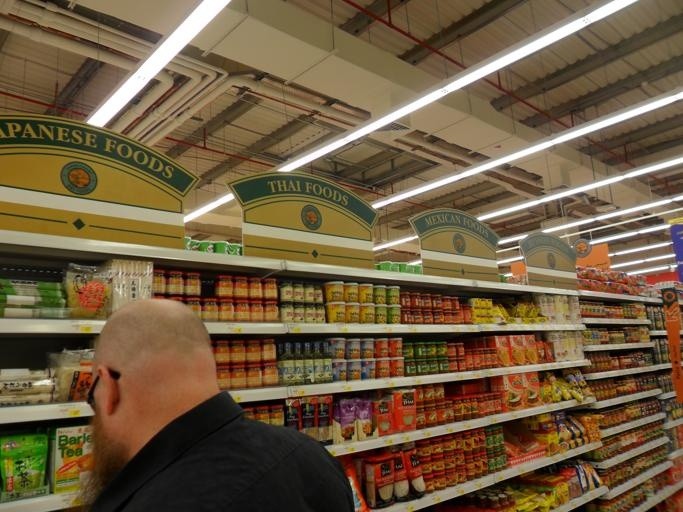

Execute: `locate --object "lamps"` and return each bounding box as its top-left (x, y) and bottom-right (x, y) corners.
top-left (83, 0), bottom-right (683, 246)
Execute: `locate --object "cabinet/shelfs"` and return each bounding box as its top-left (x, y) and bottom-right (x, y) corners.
top-left (0, 229), bottom-right (683, 512)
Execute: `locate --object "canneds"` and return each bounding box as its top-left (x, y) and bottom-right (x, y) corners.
top-left (209, 338), bottom-right (279, 390)
top-left (399, 291), bottom-right (508, 494)
top-left (240, 404), bottom-right (285, 427)
top-left (152, 269), bottom-right (325, 323)
top-left (577, 301), bottom-right (668, 512)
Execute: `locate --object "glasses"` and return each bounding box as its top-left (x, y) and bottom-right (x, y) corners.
top-left (84, 368), bottom-right (121, 414)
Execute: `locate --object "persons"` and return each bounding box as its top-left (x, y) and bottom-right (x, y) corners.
top-left (73, 298), bottom-right (355, 510)
